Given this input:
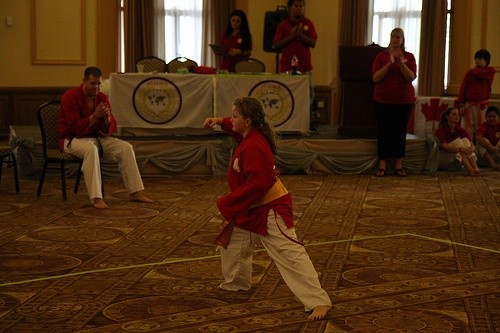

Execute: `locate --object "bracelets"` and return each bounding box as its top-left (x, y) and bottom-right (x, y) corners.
top-left (92, 114), bottom-right (99, 119)
top-left (385, 65), bottom-right (392, 69)
top-left (459, 147), bottom-right (462, 153)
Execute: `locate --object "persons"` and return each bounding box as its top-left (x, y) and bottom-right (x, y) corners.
top-left (458, 49), bottom-right (495, 145)
top-left (220, 9), bottom-right (252, 72)
top-left (203, 97), bottom-right (333, 319)
top-left (371, 28), bottom-right (417, 176)
top-left (434, 106), bottom-right (485, 176)
top-left (475, 106), bottom-right (500, 171)
top-left (273, 0), bottom-right (317, 130)
top-left (59, 67), bottom-right (153, 209)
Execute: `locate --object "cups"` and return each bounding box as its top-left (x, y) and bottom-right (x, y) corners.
top-left (137, 65), bottom-right (144, 73)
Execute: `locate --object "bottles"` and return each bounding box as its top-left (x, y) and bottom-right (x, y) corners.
top-left (291, 53), bottom-right (299, 75)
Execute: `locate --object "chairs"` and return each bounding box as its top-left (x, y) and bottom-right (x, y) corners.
top-left (36, 100), bottom-right (105, 201)
top-left (168, 57), bottom-right (198, 74)
top-left (135, 57), bottom-right (167, 73)
top-left (234, 59), bottom-right (265, 75)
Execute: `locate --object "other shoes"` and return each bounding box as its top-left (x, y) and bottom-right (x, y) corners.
top-left (394, 168), bottom-right (407, 177)
top-left (375, 168), bottom-right (387, 177)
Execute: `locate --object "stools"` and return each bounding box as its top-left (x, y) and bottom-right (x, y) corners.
top-left (0, 148), bottom-right (20, 194)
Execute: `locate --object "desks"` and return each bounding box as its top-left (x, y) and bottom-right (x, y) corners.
top-left (108, 72), bottom-right (310, 137)
